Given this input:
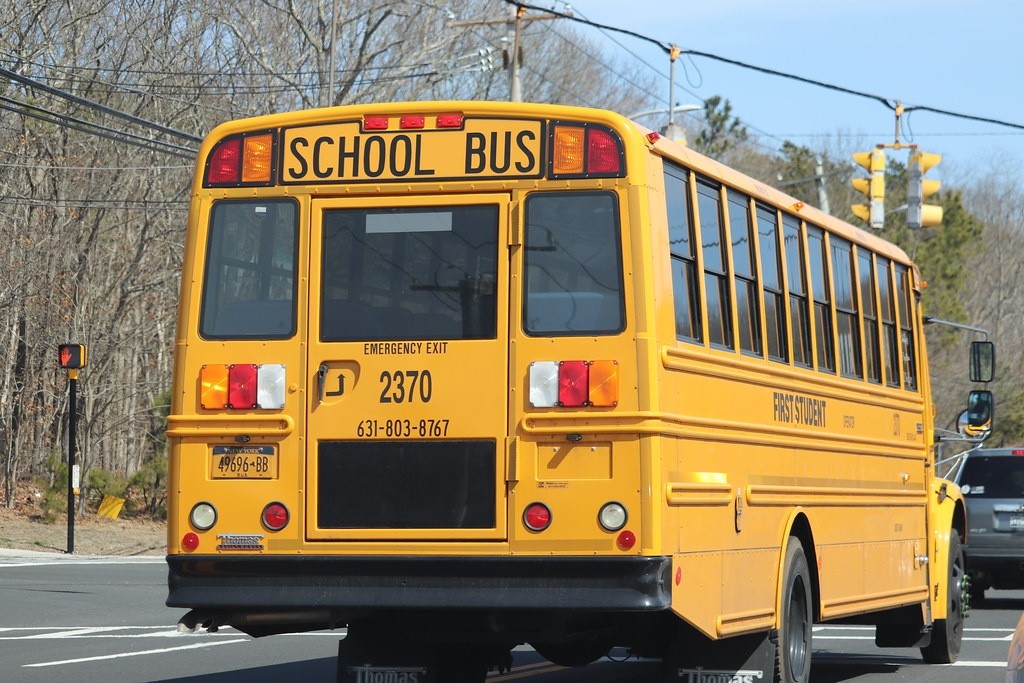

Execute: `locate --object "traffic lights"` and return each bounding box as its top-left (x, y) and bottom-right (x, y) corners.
top-left (907, 149), bottom-right (943, 230)
top-left (850, 148), bottom-right (885, 231)
top-left (58, 344), bottom-right (87, 369)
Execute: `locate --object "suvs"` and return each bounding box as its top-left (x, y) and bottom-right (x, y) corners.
top-left (952, 447), bottom-right (1024, 607)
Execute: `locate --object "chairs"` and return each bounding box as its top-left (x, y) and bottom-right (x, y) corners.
top-left (216, 297), bottom-right (464, 341)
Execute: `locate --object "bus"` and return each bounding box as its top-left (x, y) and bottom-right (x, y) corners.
top-left (165, 100), bottom-right (994, 683)
